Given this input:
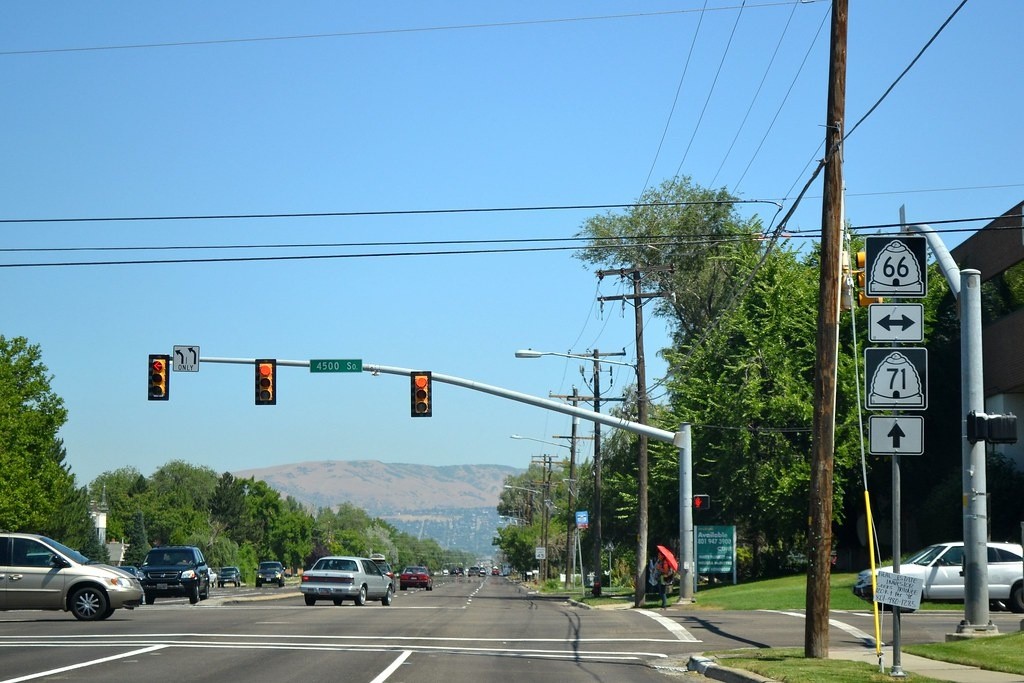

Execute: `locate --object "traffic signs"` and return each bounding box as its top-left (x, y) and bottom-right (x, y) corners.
top-left (173, 346), bottom-right (200, 371)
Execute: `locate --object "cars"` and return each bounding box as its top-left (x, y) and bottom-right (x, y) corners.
top-left (299, 556), bottom-right (395, 605)
top-left (208, 567), bottom-right (218, 584)
top-left (116, 565), bottom-right (139, 576)
top-left (443, 567), bottom-right (501, 576)
top-left (852, 542), bottom-right (1024, 613)
top-left (401, 566), bottom-right (434, 591)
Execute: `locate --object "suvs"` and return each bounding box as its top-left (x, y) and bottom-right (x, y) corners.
top-left (218, 567), bottom-right (241, 587)
top-left (254, 562), bottom-right (286, 587)
top-left (138, 545), bottom-right (210, 605)
top-left (369, 554), bottom-right (394, 579)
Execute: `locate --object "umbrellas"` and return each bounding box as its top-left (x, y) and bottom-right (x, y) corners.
top-left (657, 545), bottom-right (677, 572)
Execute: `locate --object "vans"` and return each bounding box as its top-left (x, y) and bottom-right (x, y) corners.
top-left (0, 532), bottom-right (146, 622)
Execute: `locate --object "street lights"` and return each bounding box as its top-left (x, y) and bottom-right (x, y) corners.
top-left (500, 484), bottom-right (549, 582)
top-left (516, 350), bottom-right (651, 607)
top-left (509, 435), bottom-right (575, 588)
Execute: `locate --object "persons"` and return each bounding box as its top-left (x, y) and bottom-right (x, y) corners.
top-left (656, 553), bottom-right (670, 607)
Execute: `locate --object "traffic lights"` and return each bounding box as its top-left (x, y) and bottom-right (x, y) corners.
top-left (255, 358), bottom-right (276, 405)
top-left (692, 494), bottom-right (710, 508)
top-left (148, 355), bottom-right (169, 401)
top-left (856, 251), bottom-right (882, 307)
top-left (411, 371), bottom-right (431, 417)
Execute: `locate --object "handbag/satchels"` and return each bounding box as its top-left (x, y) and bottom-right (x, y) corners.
top-left (659, 573), bottom-right (672, 586)
